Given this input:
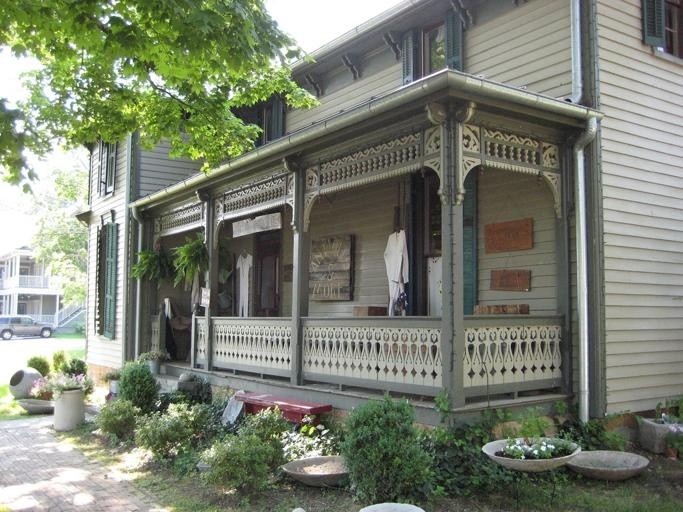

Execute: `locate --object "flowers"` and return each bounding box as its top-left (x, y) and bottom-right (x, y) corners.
top-left (665, 423), bottom-right (683, 454)
top-left (503, 436), bottom-right (571, 459)
top-left (26, 369), bottom-right (92, 400)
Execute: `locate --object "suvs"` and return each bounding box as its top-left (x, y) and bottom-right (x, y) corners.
top-left (0, 316), bottom-right (55, 340)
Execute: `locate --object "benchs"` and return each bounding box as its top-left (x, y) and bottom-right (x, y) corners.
top-left (235, 391), bottom-right (333, 426)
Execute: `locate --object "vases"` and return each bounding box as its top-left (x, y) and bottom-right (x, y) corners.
top-left (566, 450), bottom-right (650, 482)
top-left (665, 448), bottom-right (678, 460)
top-left (482, 439), bottom-right (582, 473)
top-left (51, 390), bottom-right (85, 432)
top-left (641, 417), bottom-right (670, 453)
top-left (18, 398), bottom-right (53, 414)
